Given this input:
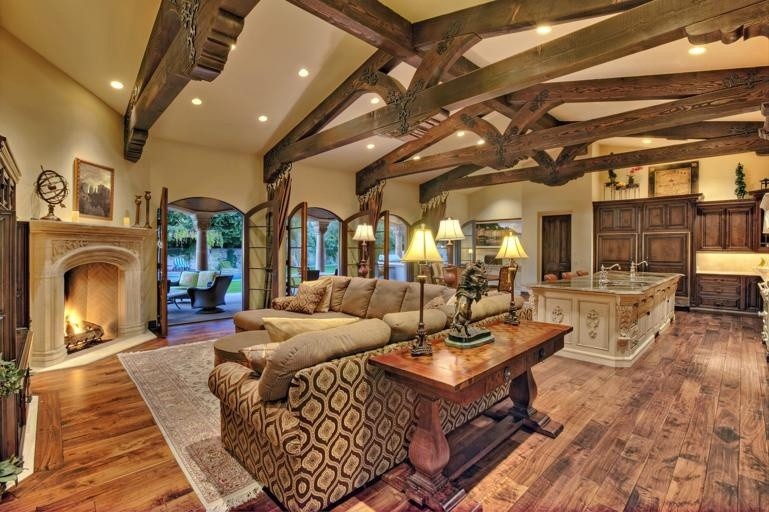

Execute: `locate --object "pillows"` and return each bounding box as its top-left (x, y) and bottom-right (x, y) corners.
top-left (288, 280), bottom-right (333, 312)
top-left (263, 317), bottom-right (358, 340)
top-left (241, 344), bottom-right (278, 369)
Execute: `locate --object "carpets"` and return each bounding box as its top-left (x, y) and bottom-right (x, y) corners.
top-left (116, 339), bottom-right (262, 511)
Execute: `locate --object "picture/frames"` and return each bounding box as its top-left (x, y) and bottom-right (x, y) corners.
top-left (74, 159), bottom-right (115, 221)
top-left (473, 218), bottom-right (523, 248)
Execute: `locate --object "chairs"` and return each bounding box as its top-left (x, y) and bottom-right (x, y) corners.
top-left (420, 263), bottom-right (518, 302)
top-left (544, 270), bottom-right (589, 286)
top-left (164, 270), bottom-right (233, 315)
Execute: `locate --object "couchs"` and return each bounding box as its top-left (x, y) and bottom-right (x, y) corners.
top-left (208, 272), bottom-right (534, 512)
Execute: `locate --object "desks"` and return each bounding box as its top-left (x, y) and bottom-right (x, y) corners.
top-left (368, 318), bottom-right (573, 512)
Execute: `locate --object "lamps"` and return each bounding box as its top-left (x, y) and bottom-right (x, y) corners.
top-left (353, 222), bottom-right (375, 277)
top-left (496, 232), bottom-right (528, 325)
top-left (436, 218), bottom-right (465, 265)
top-left (401, 224), bottom-right (444, 357)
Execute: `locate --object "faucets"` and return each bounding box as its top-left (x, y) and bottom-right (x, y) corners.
top-left (629, 260), bottom-right (649, 278)
top-left (600, 264), bottom-right (621, 283)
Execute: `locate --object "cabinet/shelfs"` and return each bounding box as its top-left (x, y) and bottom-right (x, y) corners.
top-left (636, 277), bottom-right (683, 364)
top-left (697, 202), bottom-right (752, 252)
top-left (594, 201), bottom-right (639, 233)
top-left (644, 267), bottom-right (687, 296)
top-left (596, 232), bottom-right (639, 264)
top-left (695, 274), bottom-right (744, 311)
top-left (641, 232), bottom-right (690, 264)
top-left (642, 201), bottom-right (692, 231)
